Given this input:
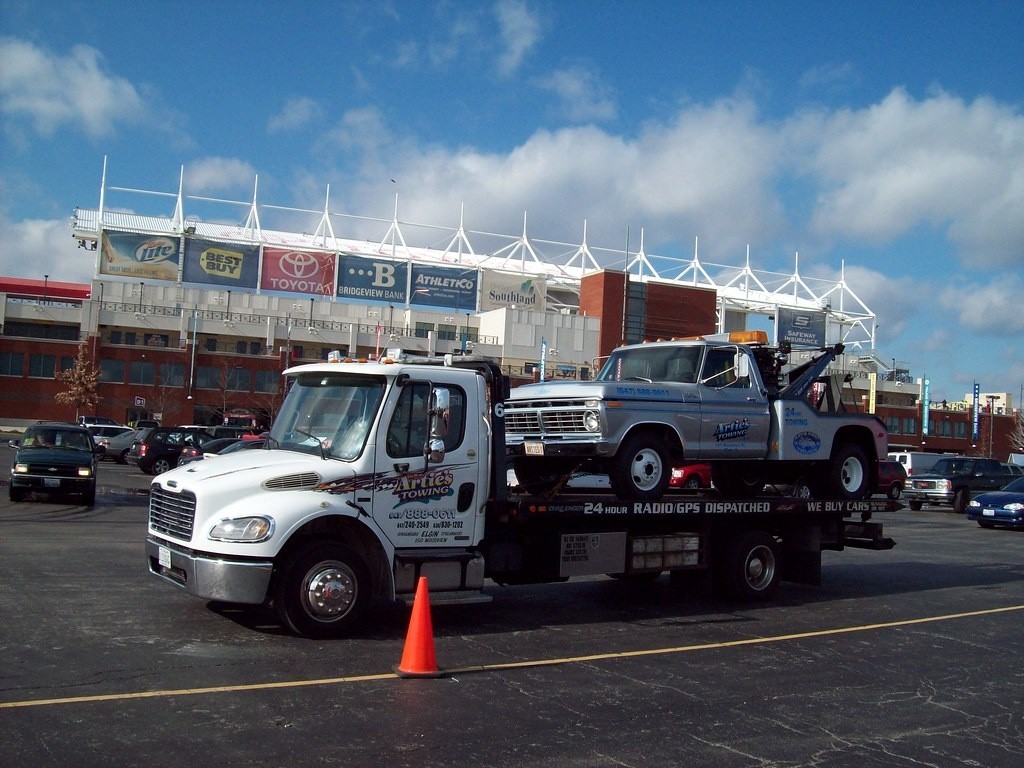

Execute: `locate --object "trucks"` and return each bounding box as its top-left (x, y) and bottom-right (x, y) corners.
top-left (144, 351), bottom-right (897, 642)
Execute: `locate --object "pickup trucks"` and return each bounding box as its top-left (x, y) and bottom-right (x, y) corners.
top-left (503, 329), bottom-right (890, 503)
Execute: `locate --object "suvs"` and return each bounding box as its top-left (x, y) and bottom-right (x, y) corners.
top-left (866, 461), bottom-right (907, 501)
top-left (901, 457), bottom-right (1021, 514)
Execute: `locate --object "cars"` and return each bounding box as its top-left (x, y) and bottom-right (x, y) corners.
top-left (177, 440), bottom-right (267, 466)
top-left (964, 476), bottom-right (1024, 531)
top-left (98, 426), bottom-right (147, 465)
top-left (173, 437), bottom-right (246, 459)
top-left (8, 421), bottom-right (100, 506)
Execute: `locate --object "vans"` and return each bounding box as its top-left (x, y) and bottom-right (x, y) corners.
top-left (82, 423), bottom-right (135, 462)
top-left (124, 426), bottom-right (218, 476)
top-left (1000, 461), bottom-right (1024, 477)
top-left (887, 451), bottom-right (957, 477)
top-left (207, 426), bottom-right (265, 438)
top-left (76, 415), bottom-right (123, 427)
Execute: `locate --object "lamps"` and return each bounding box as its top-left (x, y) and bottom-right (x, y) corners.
top-left (395, 337), bottom-right (400, 342)
top-left (230, 322), bottom-right (235, 328)
top-left (548, 349), bottom-right (555, 355)
top-left (134, 311), bottom-right (142, 320)
top-left (40, 305), bottom-right (43, 312)
top-left (32, 304), bottom-right (38, 312)
top-left (307, 326), bottom-right (315, 334)
top-left (554, 351), bottom-right (559, 355)
top-left (223, 320), bottom-right (230, 327)
top-left (472, 343), bottom-right (477, 348)
top-left (466, 340), bottom-right (472, 348)
top-left (315, 330), bottom-right (320, 335)
top-left (142, 315), bottom-right (147, 320)
top-left (390, 334), bottom-right (396, 341)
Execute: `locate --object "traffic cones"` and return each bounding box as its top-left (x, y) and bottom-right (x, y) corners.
top-left (391, 576), bottom-right (449, 680)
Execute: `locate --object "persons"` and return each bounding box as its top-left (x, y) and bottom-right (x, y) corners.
top-left (36, 432), bottom-right (51, 446)
top-left (62, 434), bottom-right (84, 446)
top-left (423, 394), bottom-right (444, 463)
